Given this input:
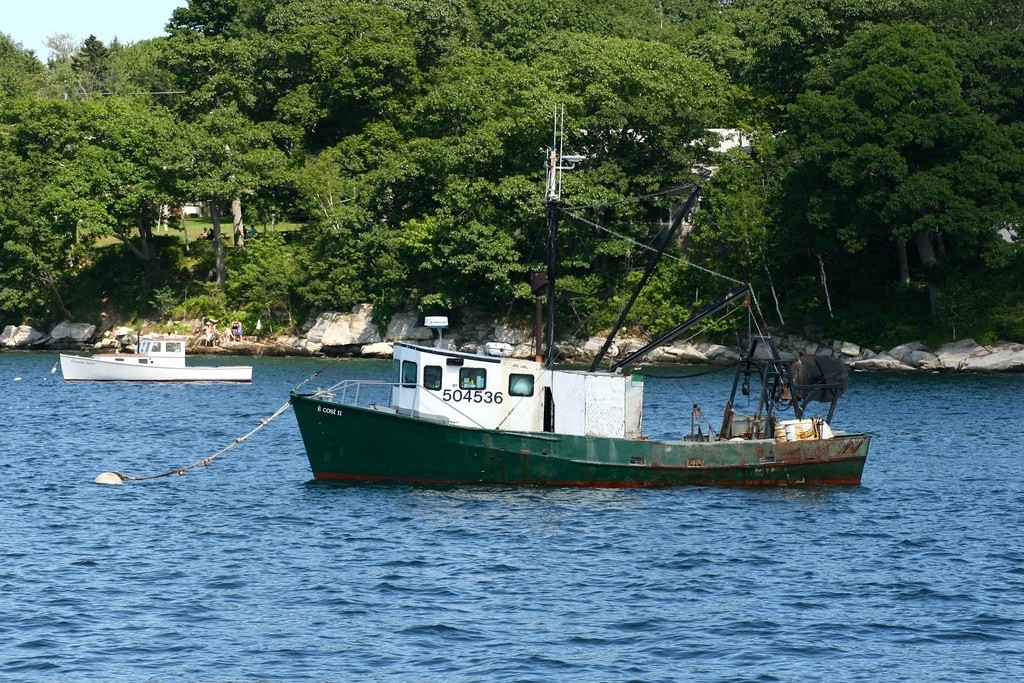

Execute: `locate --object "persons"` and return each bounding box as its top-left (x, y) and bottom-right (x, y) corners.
top-left (231, 317), bottom-right (243, 341)
top-left (223, 326), bottom-right (235, 341)
top-left (199, 227), bottom-right (215, 241)
top-left (203, 322), bottom-right (221, 347)
top-left (243, 226), bottom-right (248, 235)
top-left (245, 224), bottom-right (256, 239)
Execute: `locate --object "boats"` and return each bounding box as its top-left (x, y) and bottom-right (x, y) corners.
top-left (288, 103), bottom-right (872, 490)
top-left (60, 330), bottom-right (253, 383)
